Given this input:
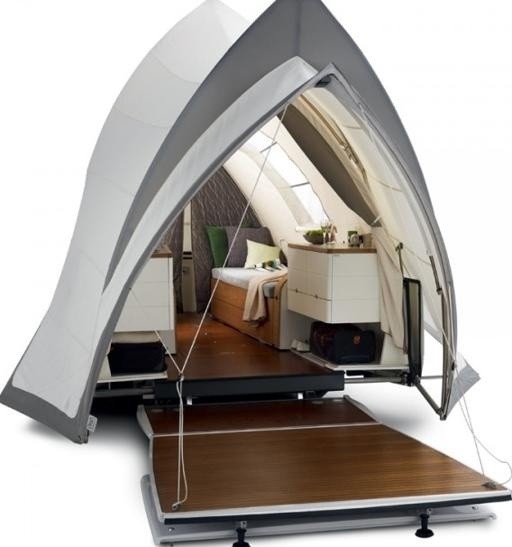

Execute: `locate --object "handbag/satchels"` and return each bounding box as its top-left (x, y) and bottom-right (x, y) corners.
top-left (309, 321), bottom-right (384, 365)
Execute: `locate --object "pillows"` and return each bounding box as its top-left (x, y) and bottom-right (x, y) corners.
top-left (244, 238), bottom-right (281, 267)
top-left (204, 221), bottom-right (252, 267)
top-left (225, 225), bottom-right (273, 267)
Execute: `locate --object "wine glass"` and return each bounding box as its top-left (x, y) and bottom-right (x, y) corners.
top-left (325, 218), bottom-right (335, 247)
top-left (319, 219), bottom-right (327, 247)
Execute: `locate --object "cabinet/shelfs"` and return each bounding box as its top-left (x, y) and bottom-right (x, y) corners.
top-left (112, 245), bottom-right (178, 333)
top-left (286, 241), bottom-right (383, 323)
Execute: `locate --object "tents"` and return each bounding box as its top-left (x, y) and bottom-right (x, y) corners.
top-left (0, 0), bottom-right (481, 445)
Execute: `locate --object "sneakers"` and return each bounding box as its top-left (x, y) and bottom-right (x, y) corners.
top-left (297, 342), bottom-right (309, 352)
top-left (291, 338), bottom-right (305, 349)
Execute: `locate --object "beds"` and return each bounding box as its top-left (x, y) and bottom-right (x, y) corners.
top-left (210, 265), bottom-right (319, 349)
